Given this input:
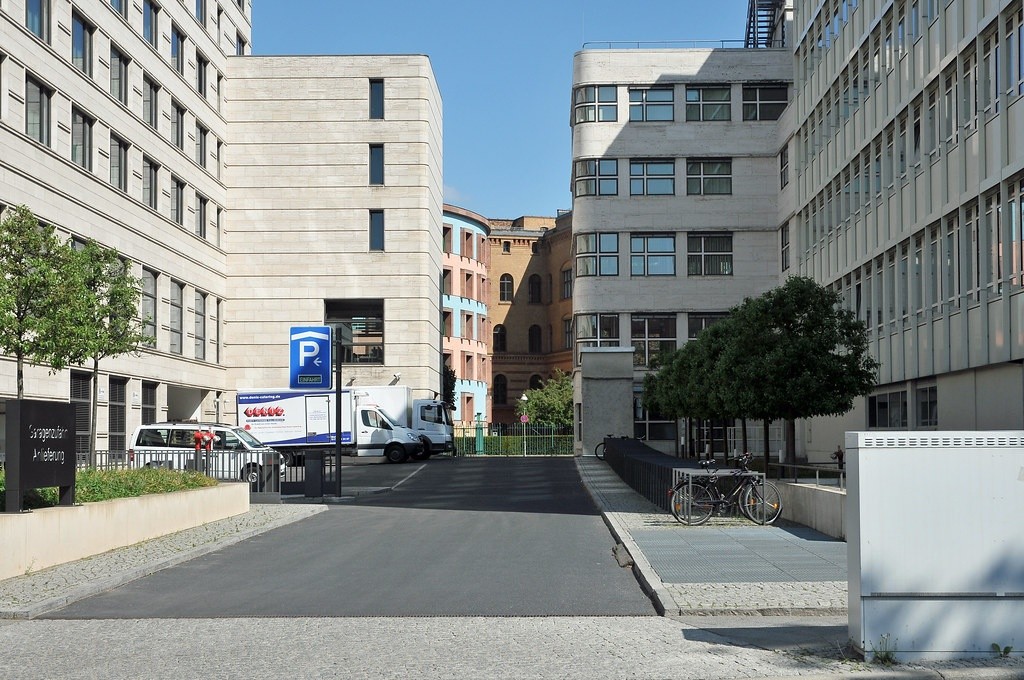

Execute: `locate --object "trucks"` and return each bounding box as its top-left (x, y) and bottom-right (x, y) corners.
top-left (342, 386), bottom-right (456, 461)
top-left (236, 387), bottom-right (424, 464)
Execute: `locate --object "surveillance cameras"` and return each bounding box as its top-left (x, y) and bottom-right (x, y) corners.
top-left (394, 372), bottom-right (402, 378)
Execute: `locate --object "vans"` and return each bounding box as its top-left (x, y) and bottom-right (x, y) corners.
top-left (129, 420), bottom-right (287, 491)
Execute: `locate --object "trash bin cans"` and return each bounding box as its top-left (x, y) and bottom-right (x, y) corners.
top-left (305, 450), bottom-right (325, 497)
top-left (263, 452), bottom-right (280, 493)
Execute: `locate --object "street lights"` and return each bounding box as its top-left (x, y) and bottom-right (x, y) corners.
top-left (520, 394), bottom-right (529, 457)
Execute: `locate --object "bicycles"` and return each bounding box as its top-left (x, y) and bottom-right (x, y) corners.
top-left (595, 434), bottom-right (646, 460)
top-left (668, 452), bottom-right (785, 525)
